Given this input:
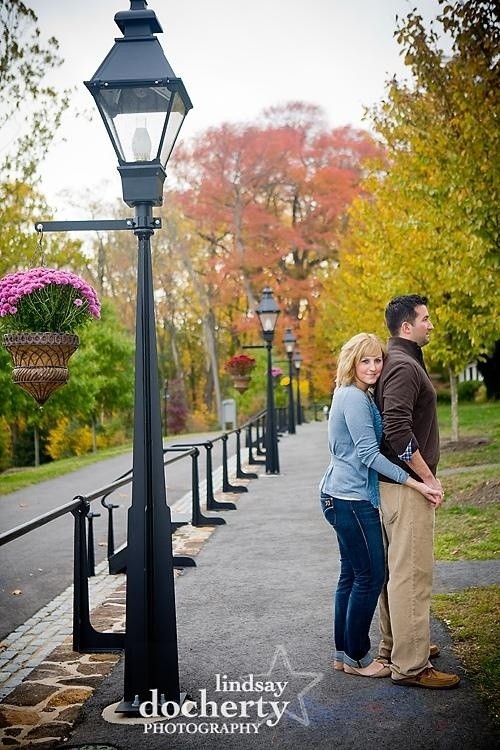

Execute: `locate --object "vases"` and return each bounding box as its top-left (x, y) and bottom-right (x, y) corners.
top-left (233, 377), bottom-right (251, 394)
top-left (2, 333), bottom-right (80, 414)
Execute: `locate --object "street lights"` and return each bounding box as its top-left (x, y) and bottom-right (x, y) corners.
top-left (83, 0), bottom-right (193, 717)
top-left (253, 288), bottom-right (303, 475)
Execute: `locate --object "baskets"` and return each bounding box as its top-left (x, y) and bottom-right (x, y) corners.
top-left (1, 328), bottom-right (77, 401)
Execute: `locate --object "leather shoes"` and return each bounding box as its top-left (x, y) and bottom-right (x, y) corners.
top-left (385, 646), bottom-right (461, 689)
top-left (333, 656), bottom-right (390, 678)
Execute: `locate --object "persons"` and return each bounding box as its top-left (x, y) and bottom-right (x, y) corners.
top-left (318, 295), bottom-right (462, 688)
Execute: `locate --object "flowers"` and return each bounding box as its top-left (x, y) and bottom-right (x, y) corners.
top-left (224, 355), bottom-right (257, 376)
top-left (0, 266), bottom-right (101, 331)
